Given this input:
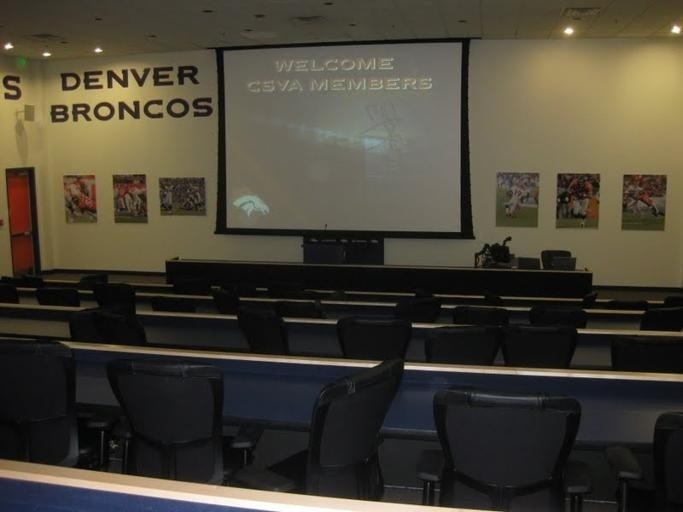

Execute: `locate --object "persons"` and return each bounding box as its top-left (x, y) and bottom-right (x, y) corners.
top-left (62, 175), bottom-right (205, 222)
top-left (497, 173), bottom-right (665, 218)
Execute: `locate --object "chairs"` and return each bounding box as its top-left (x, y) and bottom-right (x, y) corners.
top-left (0, 265), bottom-right (682, 511)
top-left (540, 250), bottom-right (575, 271)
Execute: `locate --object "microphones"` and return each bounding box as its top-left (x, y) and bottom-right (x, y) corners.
top-left (323, 224), bottom-right (328, 240)
